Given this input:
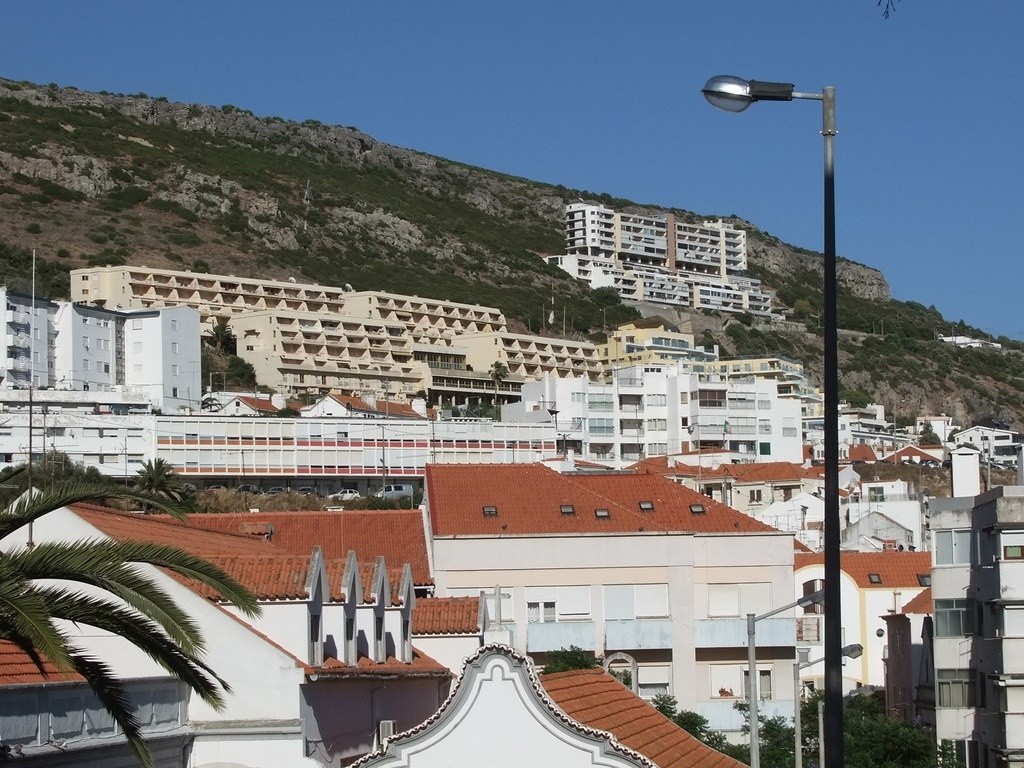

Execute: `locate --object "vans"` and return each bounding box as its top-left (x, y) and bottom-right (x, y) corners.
top-left (373, 484), bottom-right (414, 502)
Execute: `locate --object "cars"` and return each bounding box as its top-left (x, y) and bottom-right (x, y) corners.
top-left (296, 487), bottom-right (315, 497)
top-left (232, 484), bottom-right (259, 495)
top-left (327, 488), bottom-right (361, 502)
top-left (208, 485), bottom-right (228, 493)
top-left (172, 483), bottom-right (197, 495)
top-left (267, 487), bottom-right (285, 495)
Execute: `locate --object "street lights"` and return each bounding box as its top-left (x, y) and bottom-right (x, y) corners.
top-left (703, 75), bottom-right (845, 768)
top-left (792, 642), bottom-right (863, 767)
top-left (744, 587), bottom-right (824, 768)
